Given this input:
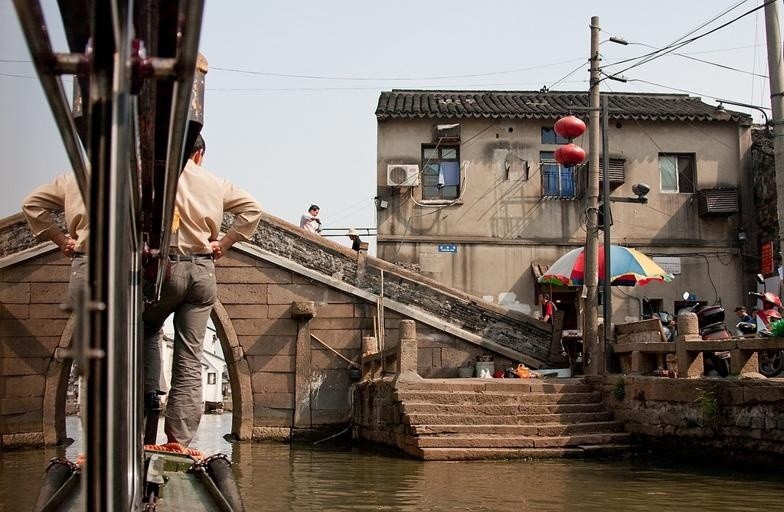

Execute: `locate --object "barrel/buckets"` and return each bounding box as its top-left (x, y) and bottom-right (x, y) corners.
top-left (475, 362), bottom-right (494, 376)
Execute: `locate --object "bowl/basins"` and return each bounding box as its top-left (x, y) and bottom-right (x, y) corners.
top-left (457, 366), bottom-right (474, 378)
top-left (477, 355), bottom-right (494, 362)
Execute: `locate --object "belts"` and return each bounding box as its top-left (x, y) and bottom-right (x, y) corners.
top-left (168, 254), bottom-right (212, 262)
top-left (72, 252), bottom-right (87, 258)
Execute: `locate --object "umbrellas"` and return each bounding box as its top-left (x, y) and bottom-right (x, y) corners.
top-left (535, 241), bottom-right (677, 290)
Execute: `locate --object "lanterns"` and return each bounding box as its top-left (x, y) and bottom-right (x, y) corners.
top-left (553, 141), bottom-right (586, 171)
top-left (552, 114), bottom-right (587, 143)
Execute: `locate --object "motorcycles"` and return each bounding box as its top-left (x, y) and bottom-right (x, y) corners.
top-left (683, 292), bottom-right (732, 376)
top-left (737, 273), bottom-right (782, 376)
top-left (641, 296), bottom-right (675, 342)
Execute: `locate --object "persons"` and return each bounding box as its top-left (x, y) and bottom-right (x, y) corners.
top-left (748, 306), bottom-right (757, 324)
top-left (299, 204), bottom-right (322, 238)
top-left (137, 132), bottom-right (264, 461)
top-left (668, 313), bottom-right (678, 343)
top-left (346, 227), bottom-right (362, 251)
top-left (651, 312), bottom-right (677, 378)
top-left (22, 155), bottom-right (90, 467)
top-left (536, 289), bottom-right (558, 324)
top-left (733, 305), bottom-right (750, 323)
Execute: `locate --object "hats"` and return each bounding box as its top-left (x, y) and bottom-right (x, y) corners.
top-left (345, 227), bottom-right (358, 236)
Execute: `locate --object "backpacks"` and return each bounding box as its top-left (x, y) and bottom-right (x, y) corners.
top-left (548, 300), bottom-right (558, 313)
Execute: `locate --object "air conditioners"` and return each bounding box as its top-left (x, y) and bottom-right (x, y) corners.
top-left (386, 164), bottom-right (420, 187)
